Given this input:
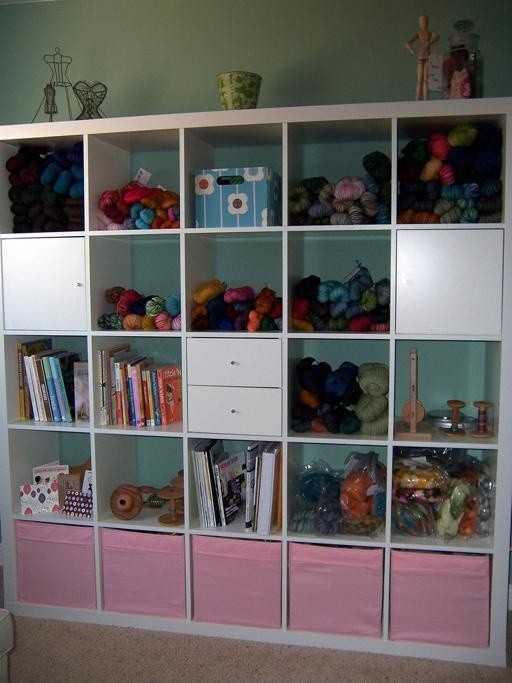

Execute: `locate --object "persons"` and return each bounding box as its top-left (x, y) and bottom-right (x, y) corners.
top-left (403, 15), bottom-right (441, 101)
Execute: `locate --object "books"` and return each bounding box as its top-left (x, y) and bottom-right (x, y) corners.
top-left (16, 337), bottom-right (182, 427)
top-left (192, 439), bottom-right (281, 534)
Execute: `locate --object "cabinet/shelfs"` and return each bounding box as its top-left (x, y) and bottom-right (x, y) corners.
top-left (1, 94), bottom-right (511, 665)
